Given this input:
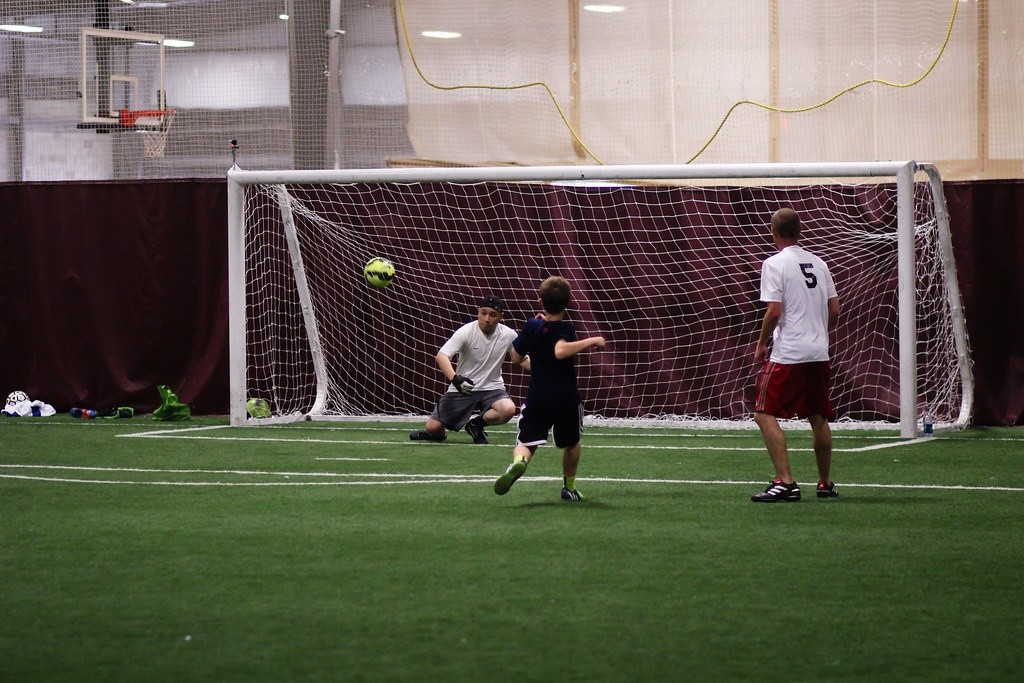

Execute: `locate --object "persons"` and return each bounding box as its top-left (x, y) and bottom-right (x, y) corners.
top-left (495, 275), bottom-right (606, 502)
top-left (750, 208), bottom-right (841, 503)
top-left (410, 296), bottom-right (530, 446)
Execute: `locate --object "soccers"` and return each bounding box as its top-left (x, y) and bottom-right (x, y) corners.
top-left (362, 256), bottom-right (396, 289)
top-left (247, 396), bottom-right (273, 419)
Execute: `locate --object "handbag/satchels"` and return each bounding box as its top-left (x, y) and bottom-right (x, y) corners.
top-left (152, 385), bottom-right (190, 422)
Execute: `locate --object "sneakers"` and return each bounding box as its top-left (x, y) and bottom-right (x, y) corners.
top-left (410, 431), bottom-right (446, 441)
top-left (816, 480), bottom-right (839, 498)
top-left (561, 487), bottom-right (587, 501)
top-left (465, 419), bottom-right (488, 444)
top-left (751, 475), bottom-right (801, 503)
top-left (494, 460), bottom-right (528, 495)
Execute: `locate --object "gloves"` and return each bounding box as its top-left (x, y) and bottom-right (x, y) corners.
top-left (450, 374), bottom-right (475, 396)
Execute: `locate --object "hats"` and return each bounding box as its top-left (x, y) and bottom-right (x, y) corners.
top-left (480, 295), bottom-right (502, 313)
